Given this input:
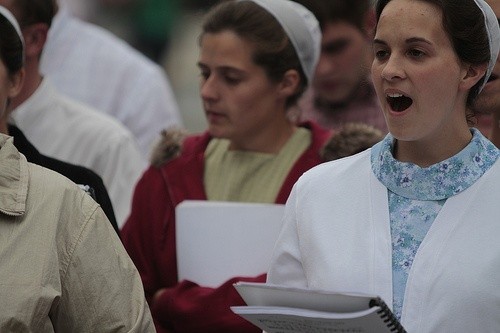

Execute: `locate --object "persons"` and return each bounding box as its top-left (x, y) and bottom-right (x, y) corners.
top-left (121, 0), bottom-right (386, 333)
top-left (0, 0), bottom-right (149, 229)
top-left (39, 13), bottom-right (185, 154)
top-left (0, 12), bottom-right (157, 333)
top-left (291, 0), bottom-right (388, 137)
top-left (267, 0), bottom-right (500, 333)
top-left (474, 0), bottom-right (500, 150)
top-left (8, 123), bottom-right (121, 238)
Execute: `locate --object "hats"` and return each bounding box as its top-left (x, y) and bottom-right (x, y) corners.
top-left (0, 5), bottom-right (25, 69)
top-left (251, 0), bottom-right (322, 92)
top-left (472, 0), bottom-right (500, 99)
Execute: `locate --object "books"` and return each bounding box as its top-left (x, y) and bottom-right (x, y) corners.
top-left (229, 279), bottom-right (407, 333)
top-left (176, 198), bottom-right (284, 287)
